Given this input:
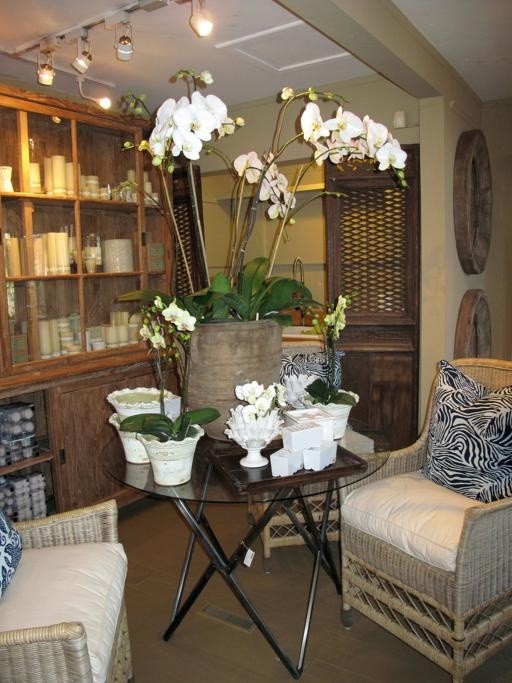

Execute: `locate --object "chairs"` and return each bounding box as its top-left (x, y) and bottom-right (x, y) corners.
top-left (333, 351), bottom-right (511, 681)
top-left (245, 334), bottom-right (379, 580)
top-left (0, 498), bottom-right (139, 683)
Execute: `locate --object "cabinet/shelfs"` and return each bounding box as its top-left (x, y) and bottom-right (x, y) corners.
top-left (0, 85), bottom-right (176, 389)
top-left (0, 382), bottom-right (62, 521)
top-left (42, 355), bottom-right (186, 514)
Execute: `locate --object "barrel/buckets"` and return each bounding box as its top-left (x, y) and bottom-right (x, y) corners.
top-left (101, 239), bottom-right (135, 274)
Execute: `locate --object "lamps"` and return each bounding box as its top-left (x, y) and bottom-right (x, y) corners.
top-left (35, 2), bottom-right (217, 91)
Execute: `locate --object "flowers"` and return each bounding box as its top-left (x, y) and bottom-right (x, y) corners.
top-left (305, 295), bottom-right (357, 408)
top-left (118, 296), bottom-right (221, 442)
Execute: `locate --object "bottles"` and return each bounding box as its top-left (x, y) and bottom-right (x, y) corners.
top-left (30, 154), bottom-right (160, 205)
top-left (21, 313), bottom-right (83, 357)
top-left (1, 488), bottom-right (14, 515)
top-left (4, 228), bottom-right (73, 277)
top-left (0, 408), bottom-right (35, 437)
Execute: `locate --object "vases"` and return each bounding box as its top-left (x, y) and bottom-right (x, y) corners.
top-left (183, 320), bottom-right (282, 441)
top-left (102, 385), bottom-right (355, 488)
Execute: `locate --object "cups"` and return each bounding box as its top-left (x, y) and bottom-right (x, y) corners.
top-left (86, 308), bottom-right (142, 352)
top-left (84, 231), bottom-right (134, 273)
top-left (0, 164), bottom-right (15, 193)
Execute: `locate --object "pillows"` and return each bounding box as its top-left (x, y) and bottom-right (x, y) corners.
top-left (421, 359), bottom-right (510, 503)
top-left (278, 349), bottom-right (347, 406)
top-left (0, 510), bottom-right (23, 601)
